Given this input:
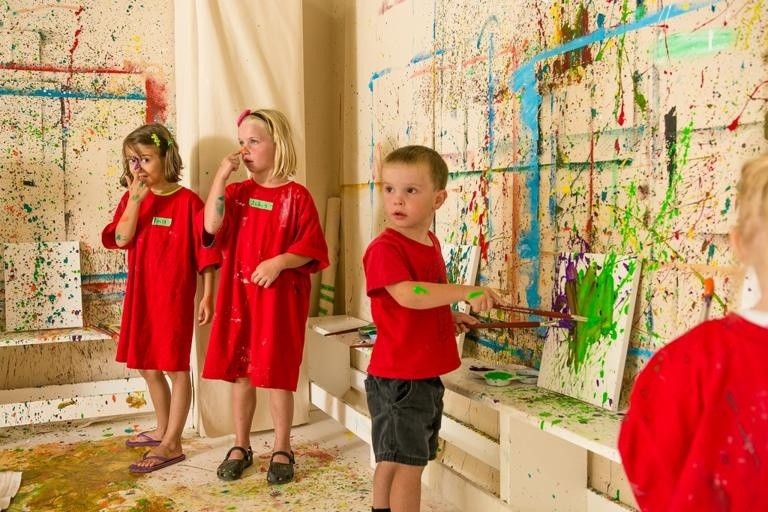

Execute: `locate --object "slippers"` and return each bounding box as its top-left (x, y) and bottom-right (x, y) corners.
top-left (128, 449), bottom-right (185, 473)
top-left (125, 430), bottom-right (162, 446)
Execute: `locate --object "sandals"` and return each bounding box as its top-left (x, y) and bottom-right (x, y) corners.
top-left (267, 450), bottom-right (295, 485)
top-left (217, 446), bottom-right (253, 481)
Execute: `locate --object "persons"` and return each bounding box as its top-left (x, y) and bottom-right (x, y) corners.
top-left (361, 145), bottom-right (508, 512)
top-left (617, 153), bottom-right (767, 512)
top-left (100, 122), bottom-right (223, 474)
top-left (199, 108), bottom-right (330, 484)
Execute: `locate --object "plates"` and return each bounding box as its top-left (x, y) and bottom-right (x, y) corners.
top-left (469, 366), bottom-right (538, 387)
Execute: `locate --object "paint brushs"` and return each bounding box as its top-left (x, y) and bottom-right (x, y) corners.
top-left (462, 299), bottom-right (588, 328)
